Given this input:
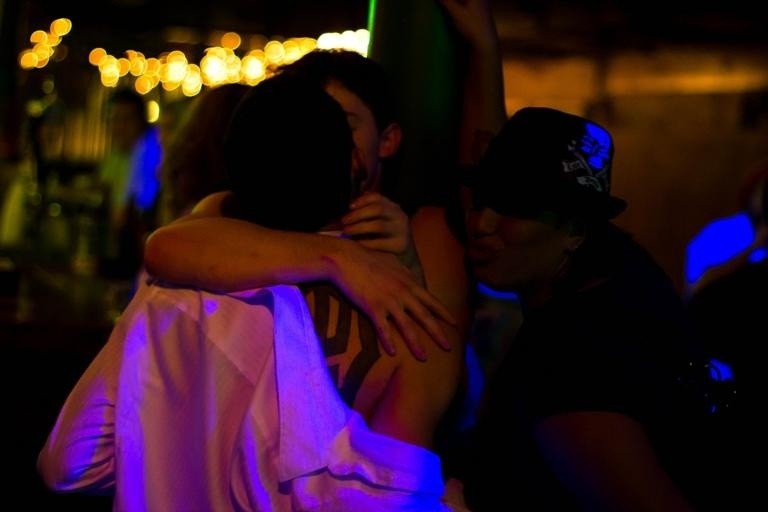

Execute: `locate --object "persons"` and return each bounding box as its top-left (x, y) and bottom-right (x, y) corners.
top-left (35, 46), bottom-right (768, 512)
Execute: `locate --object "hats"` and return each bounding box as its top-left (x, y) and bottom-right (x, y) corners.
top-left (459, 107), bottom-right (628, 220)
